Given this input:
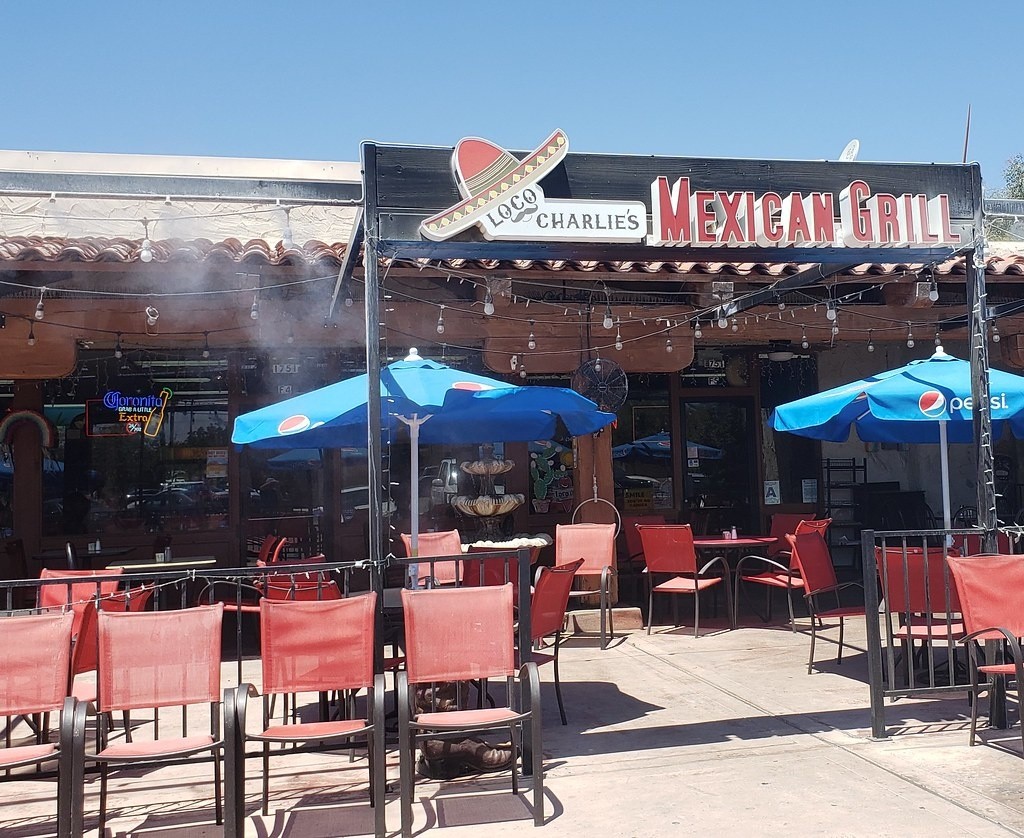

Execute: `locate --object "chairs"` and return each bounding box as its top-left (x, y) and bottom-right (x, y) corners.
top-left (0, 505), bottom-right (1024, 838)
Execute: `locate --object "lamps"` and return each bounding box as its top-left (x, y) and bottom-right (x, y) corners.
top-left (767, 339), bottom-right (793, 361)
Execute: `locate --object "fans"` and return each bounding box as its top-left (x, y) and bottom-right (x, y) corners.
top-left (570, 358), bottom-right (629, 438)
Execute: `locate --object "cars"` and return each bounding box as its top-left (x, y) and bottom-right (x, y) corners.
top-left (44, 478), bottom-right (261, 531)
top-left (338, 457), bottom-right (505, 523)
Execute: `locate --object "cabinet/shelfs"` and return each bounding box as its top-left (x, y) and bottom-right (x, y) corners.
top-left (817, 457), bottom-right (867, 576)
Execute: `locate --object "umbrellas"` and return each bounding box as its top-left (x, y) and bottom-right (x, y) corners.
top-left (266, 447), bottom-right (368, 470)
top-left (612, 429), bottom-right (723, 462)
top-left (232, 347), bottom-right (616, 590)
top-left (766, 346), bottom-right (1024, 547)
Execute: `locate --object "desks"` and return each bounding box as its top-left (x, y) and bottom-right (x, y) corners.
top-left (31, 545), bottom-right (137, 570)
top-left (104, 554), bottom-right (216, 611)
top-left (693, 534), bottom-right (779, 623)
